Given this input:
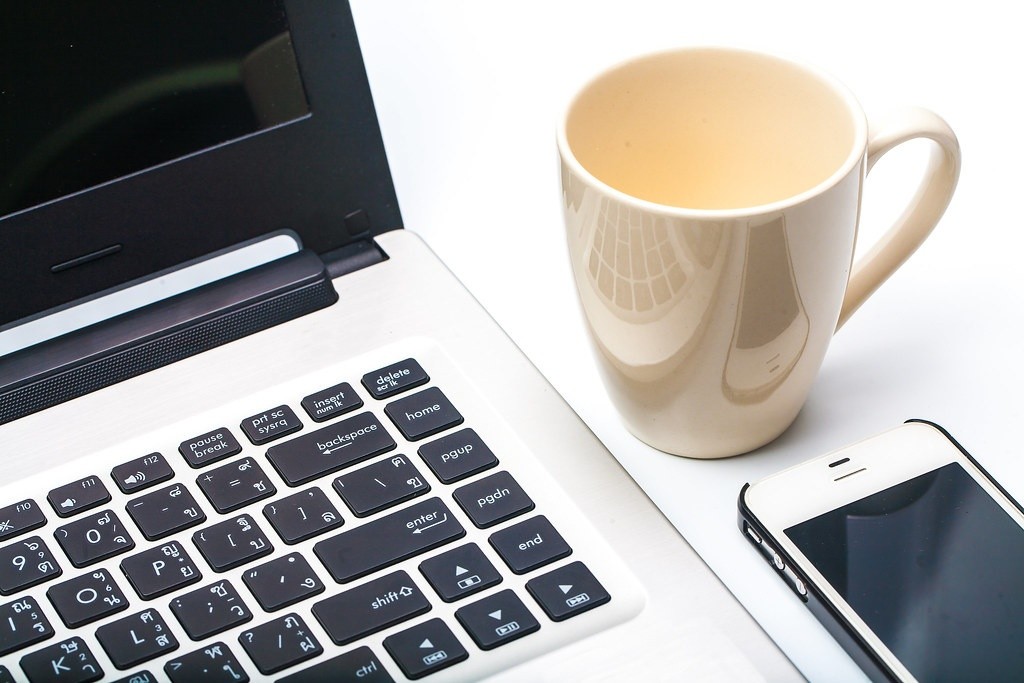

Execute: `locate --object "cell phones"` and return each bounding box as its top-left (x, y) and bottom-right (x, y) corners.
top-left (735, 417), bottom-right (1023, 683)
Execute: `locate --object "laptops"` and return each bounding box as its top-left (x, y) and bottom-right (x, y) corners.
top-left (0, 0), bottom-right (813, 683)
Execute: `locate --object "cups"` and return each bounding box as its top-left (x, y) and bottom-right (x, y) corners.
top-left (556, 48), bottom-right (960, 460)
